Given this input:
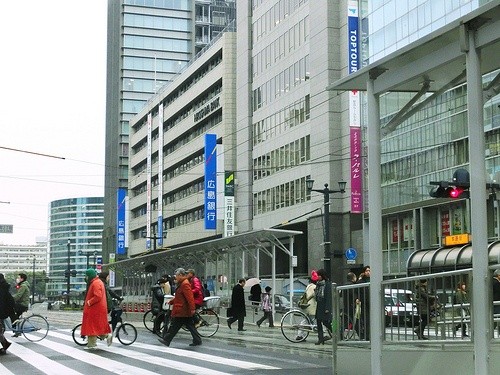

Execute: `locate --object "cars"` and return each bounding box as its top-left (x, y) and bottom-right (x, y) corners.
top-left (384, 288), bottom-right (423, 328)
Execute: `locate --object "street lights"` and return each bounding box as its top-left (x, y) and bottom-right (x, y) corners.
top-left (306, 176), bottom-right (347, 284)
top-left (31, 254), bottom-right (36, 305)
top-left (63, 238), bottom-right (77, 308)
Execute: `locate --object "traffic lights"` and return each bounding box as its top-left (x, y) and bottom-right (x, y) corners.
top-left (429, 186), bottom-right (460, 198)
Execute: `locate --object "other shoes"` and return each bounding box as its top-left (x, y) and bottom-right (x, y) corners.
top-left (237, 328), bottom-right (247, 332)
top-left (83, 344), bottom-right (98, 350)
top-left (106, 333), bottom-right (113, 347)
top-left (256, 320), bottom-right (261, 328)
top-left (296, 336), bottom-right (306, 342)
top-left (158, 338), bottom-right (170, 347)
top-left (188, 337), bottom-right (202, 346)
top-left (226, 319), bottom-right (232, 330)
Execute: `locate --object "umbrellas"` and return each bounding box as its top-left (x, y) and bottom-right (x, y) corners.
top-left (242, 278), bottom-right (261, 288)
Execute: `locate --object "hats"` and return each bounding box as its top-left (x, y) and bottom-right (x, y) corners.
top-left (84, 267), bottom-right (96, 283)
top-left (311, 271), bottom-right (319, 280)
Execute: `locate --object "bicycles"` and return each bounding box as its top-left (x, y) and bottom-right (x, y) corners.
top-left (3, 307), bottom-right (49, 342)
top-left (280, 302), bottom-right (355, 343)
top-left (71, 297), bottom-right (138, 346)
top-left (143, 299), bottom-right (219, 338)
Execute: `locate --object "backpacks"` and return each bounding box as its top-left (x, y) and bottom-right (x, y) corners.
top-left (298, 284), bottom-right (315, 309)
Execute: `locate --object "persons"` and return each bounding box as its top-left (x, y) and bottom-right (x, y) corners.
top-left (255, 286), bottom-right (274, 329)
top-left (452, 267), bottom-right (500, 339)
top-left (250, 285), bottom-right (263, 315)
top-left (96, 270), bottom-right (124, 341)
top-left (4, 273), bottom-right (30, 338)
top-left (0, 272), bottom-right (18, 355)
top-left (413, 279), bottom-right (440, 341)
top-left (294, 264), bottom-right (377, 345)
top-left (82, 267), bottom-right (111, 349)
top-left (226, 277), bottom-right (248, 332)
top-left (150, 267), bottom-right (210, 347)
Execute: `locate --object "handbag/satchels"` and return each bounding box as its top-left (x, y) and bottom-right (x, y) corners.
top-left (248, 295), bottom-right (253, 299)
top-left (226, 307), bottom-right (233, 317)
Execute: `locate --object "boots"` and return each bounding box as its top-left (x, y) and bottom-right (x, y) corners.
top-left (315, 331), bottom-right (325, 345)
